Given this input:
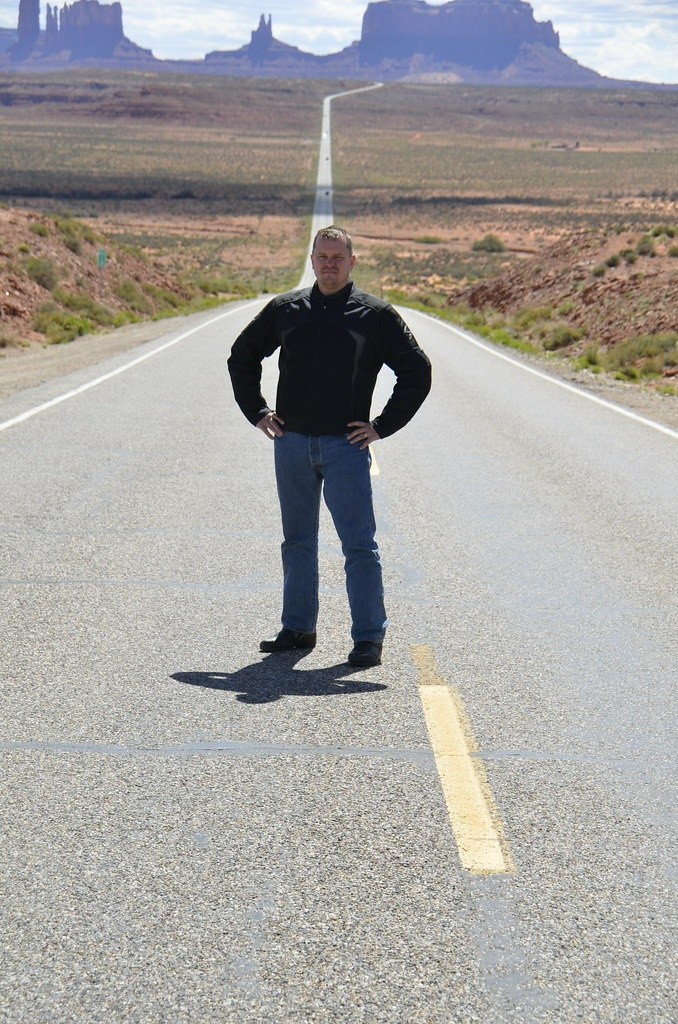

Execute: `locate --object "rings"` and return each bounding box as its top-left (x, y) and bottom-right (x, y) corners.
top-left (365, 434), bottom-right (367, 437)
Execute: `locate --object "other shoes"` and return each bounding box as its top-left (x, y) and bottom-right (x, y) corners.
top-left (260, 627), bottom-right (316, 652)
top-left (348, 641), bottom-right (382, 666)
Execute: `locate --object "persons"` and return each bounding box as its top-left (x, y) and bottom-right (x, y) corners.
top-left (227, 225), bottom-right (432, 666)
